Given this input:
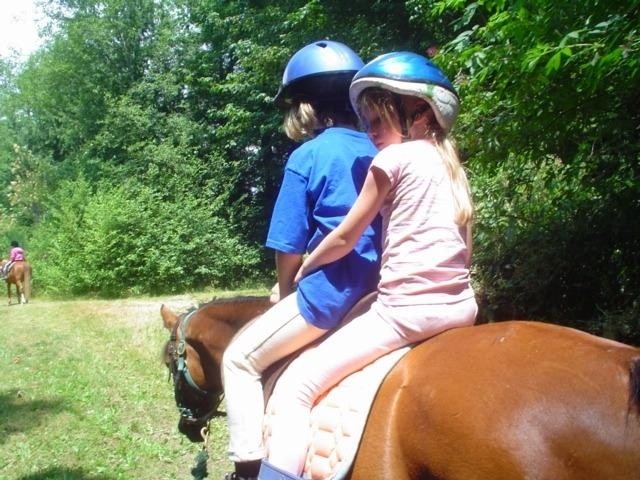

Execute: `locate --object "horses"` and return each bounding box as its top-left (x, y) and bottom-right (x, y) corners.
top-left (159, 292), bottom-right (640, 480)
top-left (0, 258), bottom-right (33, 304)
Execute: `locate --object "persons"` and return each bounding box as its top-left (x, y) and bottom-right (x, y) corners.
top-left (2, 240), bottom-right (24, 280)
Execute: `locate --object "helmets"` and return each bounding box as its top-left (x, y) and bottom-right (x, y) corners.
top-left (11, 241), bottom-right (18, 246)
top-left (272, 40), bottom-right (460, 134)
